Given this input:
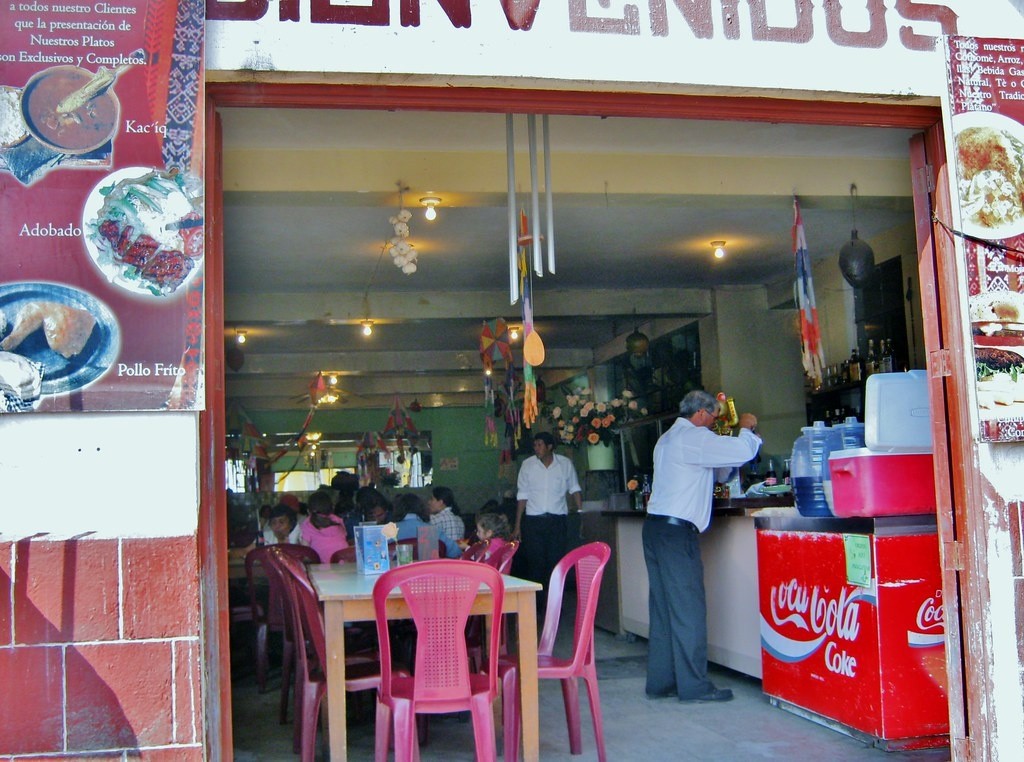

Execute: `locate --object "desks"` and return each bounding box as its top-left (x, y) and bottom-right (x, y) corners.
top-left (306, 560), bottom-right (544, 762)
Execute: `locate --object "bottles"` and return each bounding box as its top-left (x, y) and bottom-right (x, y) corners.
top-left (630, 474), bottom-right (651, 510)
top-left (764, 458), bottom-right (777, 488)
top-left (819, 337), bottom-right (909, 427)
top-left (791, 415), bottom-right (864, 518)
top-left (747, 463), bottom-right (761, 483)
top-left (782, 459), bottom-right (791, 485)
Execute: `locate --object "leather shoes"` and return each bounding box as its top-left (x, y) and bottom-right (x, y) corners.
top-left (680, 687), bottom-right (733, 705)
top-left (646, 684), bottom-right (680, 700)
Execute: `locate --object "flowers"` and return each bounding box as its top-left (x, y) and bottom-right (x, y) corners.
top-left (550, 384), bottom-right (644, 447)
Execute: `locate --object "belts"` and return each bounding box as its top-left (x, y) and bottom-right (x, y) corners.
top-left (646, 513), bottom-right (700, 535)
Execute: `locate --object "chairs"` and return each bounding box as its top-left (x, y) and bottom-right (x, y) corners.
top-left (231, 531), bottom-right (613, 762)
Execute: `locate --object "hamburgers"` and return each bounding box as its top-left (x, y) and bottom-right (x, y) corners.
top-left (968, 289), bottom-right (1024, 421)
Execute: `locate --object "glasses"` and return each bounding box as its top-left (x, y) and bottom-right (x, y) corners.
top-left (698, 409), bottom-right (717, 421)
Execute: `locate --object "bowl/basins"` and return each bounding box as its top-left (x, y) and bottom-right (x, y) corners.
top-left (21, 64), bottom-right (121, 155)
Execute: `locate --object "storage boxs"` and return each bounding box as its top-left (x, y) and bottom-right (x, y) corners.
top-left (830, 369), bottom-right (937, 517)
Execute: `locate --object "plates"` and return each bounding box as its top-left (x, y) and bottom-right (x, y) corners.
top-left (970, 290), bottom-right (1024, 343)
top-left (971, 346), bottom-right (1024, 420)
top-left (81, 166), bottom-right (205, 296)
top-left (0, 278), bottom-right (121, 401)
top-left (949, 111), bottom-right (1024, 239)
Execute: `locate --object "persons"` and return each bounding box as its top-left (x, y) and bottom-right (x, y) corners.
top-left (228, 485), bottom-right (515, 658)
top-left (642, 391), bottom-right (763, 704)
top-left (512, 431), bottom-right (586, 618)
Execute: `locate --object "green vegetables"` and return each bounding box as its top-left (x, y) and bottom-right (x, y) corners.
top-left (84, 172), bottom-right (190, 297)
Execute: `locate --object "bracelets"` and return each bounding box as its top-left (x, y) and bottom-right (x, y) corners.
top-left (578, 510), bottom-right (584, 513)
